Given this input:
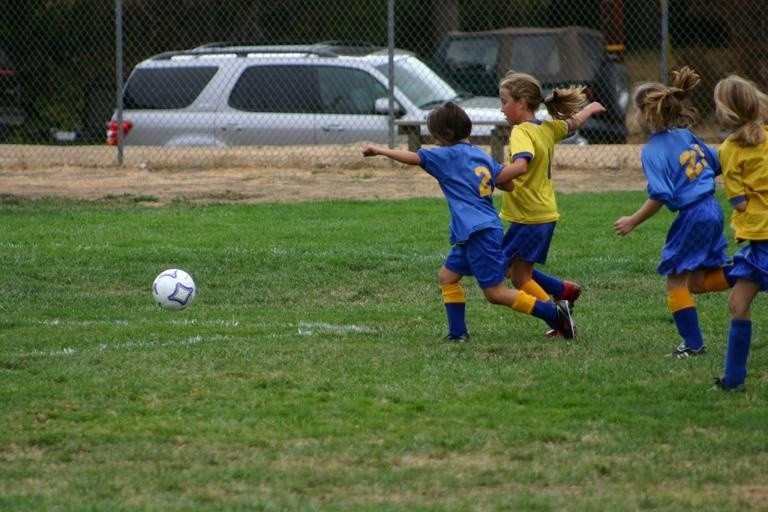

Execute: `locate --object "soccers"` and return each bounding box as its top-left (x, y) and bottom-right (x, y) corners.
top-left (152, 269), bottom-right (195, 311)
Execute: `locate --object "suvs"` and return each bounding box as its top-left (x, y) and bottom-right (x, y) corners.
top-left (430, 26), bottom-right (631, 143)
top-left (107, 37), bottom-right (587, 146)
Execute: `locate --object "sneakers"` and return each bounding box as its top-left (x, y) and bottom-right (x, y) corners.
top-left (544, 280), bottom-right (582, 342)
top-left (705, 376), bottom-right (747, 397)
top-left (664, 341), bottom-right (706, 360)
top-left (442, 331), bottom-right (469, 342)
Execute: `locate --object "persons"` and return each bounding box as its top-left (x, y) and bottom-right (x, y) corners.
top-left (361, 101), bottom-right (577, 341)
top-left (494, 72), bottom-right (607, 337)
top-left (709, 75), bottom-right (768, 393)
top-left (614, 65), bottom-right (738, 359)
